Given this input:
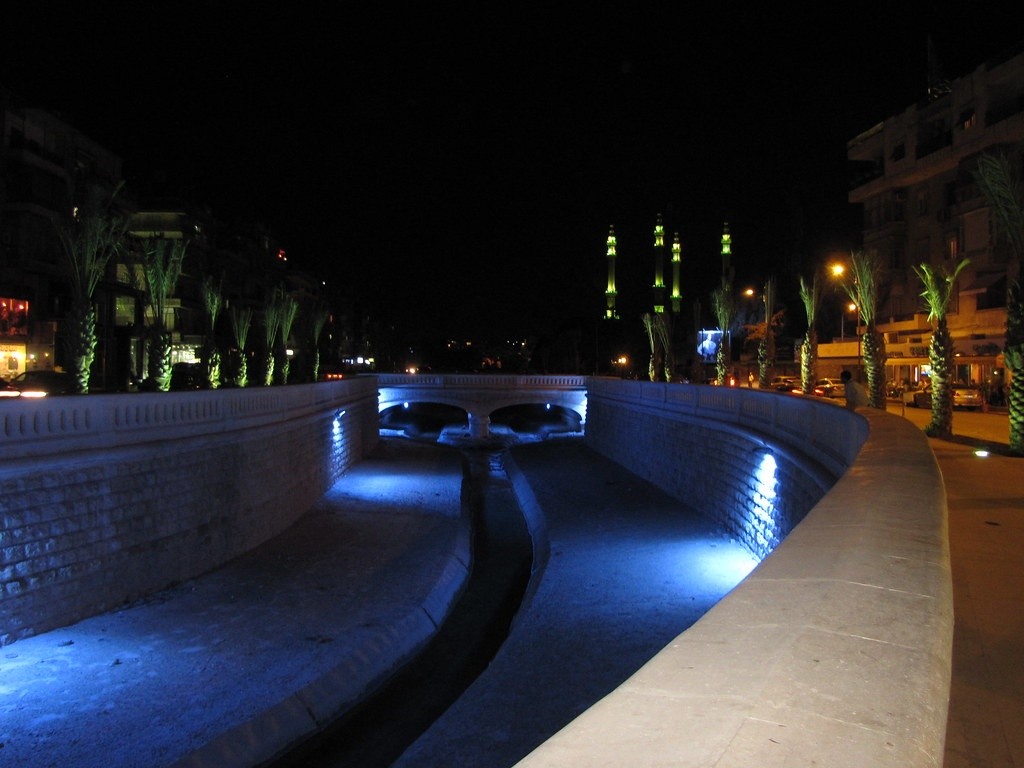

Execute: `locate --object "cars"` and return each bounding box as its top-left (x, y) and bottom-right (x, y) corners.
top-left (815, 377), bottom-right (847, 399)
top-left (902, 381), bottom-right (983, 410)
top-left (771, 375), bottom-right (798, 392)
top-left (0, 370), bottom-right (72, 401)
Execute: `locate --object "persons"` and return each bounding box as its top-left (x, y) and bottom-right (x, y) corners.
top-left (841, 371), bottom-right (872, 407)
top-left (958, 379), bottom-right (1011, 408)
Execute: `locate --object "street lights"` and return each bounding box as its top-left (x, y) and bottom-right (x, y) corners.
top-left (828, 262), bottom-right (862, 383)
